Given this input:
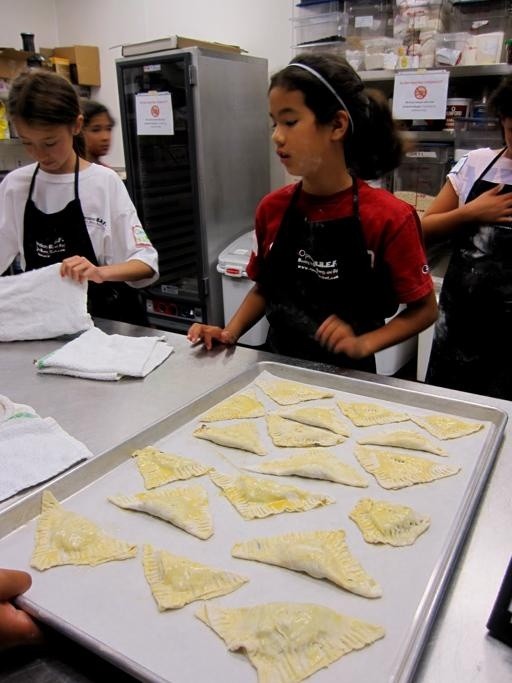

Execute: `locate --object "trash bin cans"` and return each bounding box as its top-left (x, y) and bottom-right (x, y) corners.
top-left (216, 228), bottom-right (270, 346)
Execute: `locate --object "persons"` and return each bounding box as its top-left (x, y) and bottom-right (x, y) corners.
top-left (1, 568), bottom-right (42, 644)
top-left (0, 63), bottom-right (164, 326)
top-left (417, 77), bottom-right (511, 403)
top-left (185, 48), bottom-right (439, 373)
top-left (76, 94), bottom-right (118, 166)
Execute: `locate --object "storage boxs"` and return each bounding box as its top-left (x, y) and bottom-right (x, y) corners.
top-left (0, 43), bottom-right (101, 88)
top-left (287, 0), bottom-right (474, 71)
